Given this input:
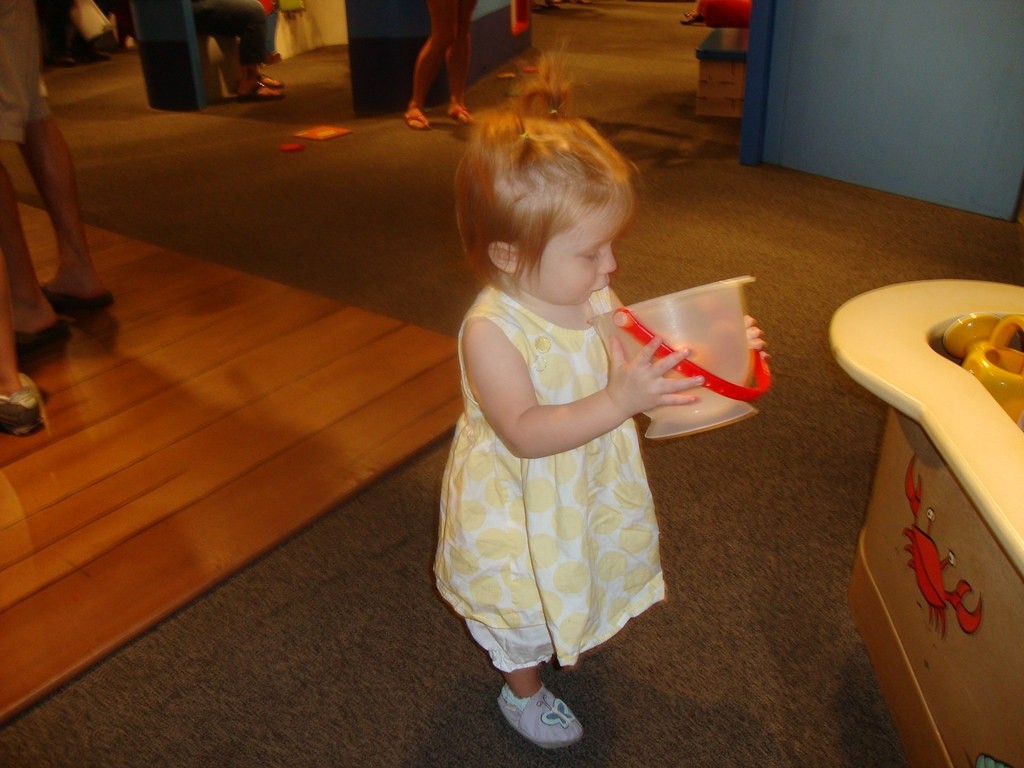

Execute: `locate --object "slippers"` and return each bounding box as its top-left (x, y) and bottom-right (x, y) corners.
top-left (41, 281), bottom-right (112, 310)
top-left (448, 111), bottom-right (474, 125)
top-left (15, 318), bottom-right (68, 355)
top-left (238, 81), bottom-right (283, 101)
top-left (256, 74), bottom-right (284, 88)
top-left (404, 111), bottom-right (429, 129)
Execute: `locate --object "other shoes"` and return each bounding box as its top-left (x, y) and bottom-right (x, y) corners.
top-left (497, 680), bottom-right (584, 748)
top-left (46, 49), bottom-right (76, 65)
top-left (71, 49), bottom-right (103, 61)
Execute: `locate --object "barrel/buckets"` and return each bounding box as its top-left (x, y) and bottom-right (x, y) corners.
top-left (589, 276), bottom-right (771, 439)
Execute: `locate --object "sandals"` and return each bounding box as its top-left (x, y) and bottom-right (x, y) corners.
top-left (681, 12), bottom-right (704, 25)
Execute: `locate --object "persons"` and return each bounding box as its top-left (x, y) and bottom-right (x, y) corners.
top-left (434, 46), bottom-right (768, 747)
top-left (191, 0), bottom-right (287, 101)
top-left (0, 0), bottom-right (112, 436)
top-left (403, 0), bottom-right (477, 130)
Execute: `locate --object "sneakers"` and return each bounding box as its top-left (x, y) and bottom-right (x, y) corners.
top-left (0, 372), bottom-right (44, 436)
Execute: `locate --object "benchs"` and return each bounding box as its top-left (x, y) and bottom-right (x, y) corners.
top-left (694, 25), bottom-right (748, 121)
